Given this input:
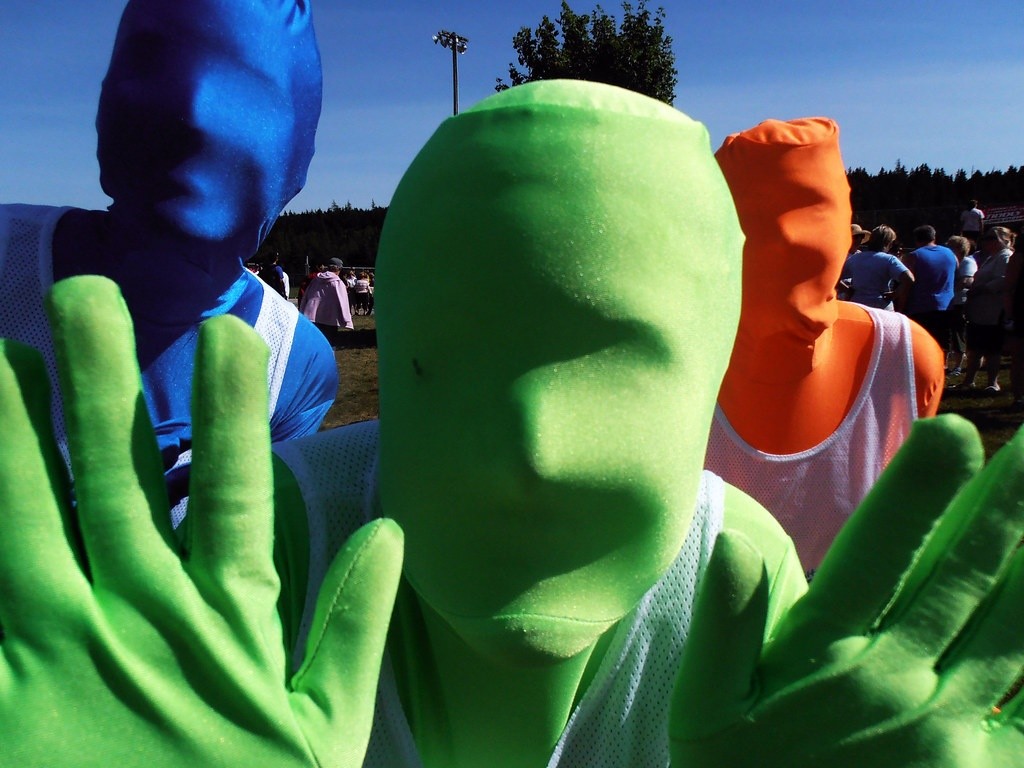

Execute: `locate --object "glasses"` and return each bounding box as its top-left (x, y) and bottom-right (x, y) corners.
top-left (984, 235), bottom-right (997, 241)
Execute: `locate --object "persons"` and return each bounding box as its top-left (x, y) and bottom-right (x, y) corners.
top-left (700, 117), bottom-right (945, 585)
top-left (960, 200), bottom-right (985, 240)
top-left (259, 250), bottom-right (285, 296)
top-left (345, 270), bottom-right (375, 316)
top-left (0, 0), bottom-right (339, 530)
top-left (298, 257), bottom-right (354, 351)
top-left (835, 224), bottom-right (1024, 392)
top-left (0, 79), bottom-right (1024, 768)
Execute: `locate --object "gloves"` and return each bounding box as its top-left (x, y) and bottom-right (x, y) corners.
top-left (669, 413), bottom-right (1024, 768)
top-left (0, 275), bottom-right (406, 768)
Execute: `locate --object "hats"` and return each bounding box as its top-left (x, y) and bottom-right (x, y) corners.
top-left (851, 224), bottom-right (871, 244)
top-left (328, 258), bottom-right (343, 266)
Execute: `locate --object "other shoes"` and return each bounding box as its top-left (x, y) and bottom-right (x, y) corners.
top-left (947, 365), bottom-right (962, 376)
top-left (350, 311), bottom-right (374, 316)
top-left (1015, 395), bottom-right (1024, 403)
top-left (953, 382), bottom-right (976, 392)
top-left (984, 382), bottom-right (1001, 395)
